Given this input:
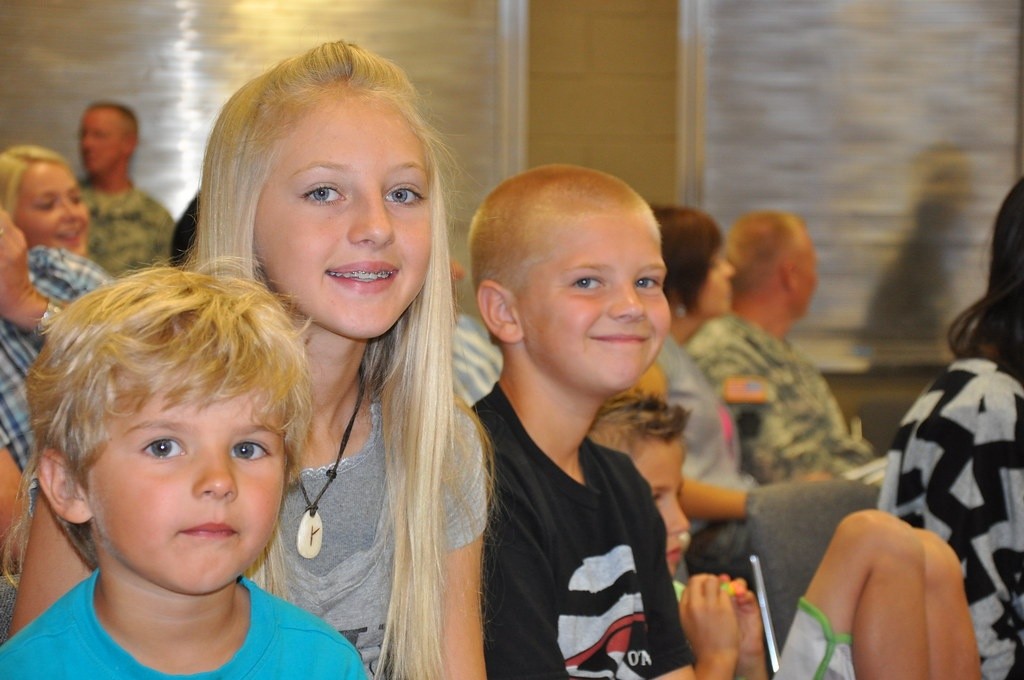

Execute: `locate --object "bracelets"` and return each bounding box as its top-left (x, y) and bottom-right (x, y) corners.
top-left (38, 296), bottom-right (63, 331)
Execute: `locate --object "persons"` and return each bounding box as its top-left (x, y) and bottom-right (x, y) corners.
top-left (587, 391), bottom-right (982, 680)
top-left (0, 144), bottom-right (91, 255)
top-left (10, 40), bottom-right (488, 680)
top-left (77, 101), bottom-right (175, 279)
top-left (0, 207), bottom-right (117, 576)
top-left (0, 267), bottom-right (369, 680)
top-left (467, 163), bottom-right (697, 680)
top-left (877, 176), bottom-right (1024, 680)
top-left (653, 205), bottom-right (834, 578)
top-left (685, 211), bottom-right (874, 484)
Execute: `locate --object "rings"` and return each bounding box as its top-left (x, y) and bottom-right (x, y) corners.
top-left (0, 228), bottom-right (4, 234)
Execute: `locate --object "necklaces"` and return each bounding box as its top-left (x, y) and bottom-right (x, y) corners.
top-left (294, 363), bottom-right (367, 559)
